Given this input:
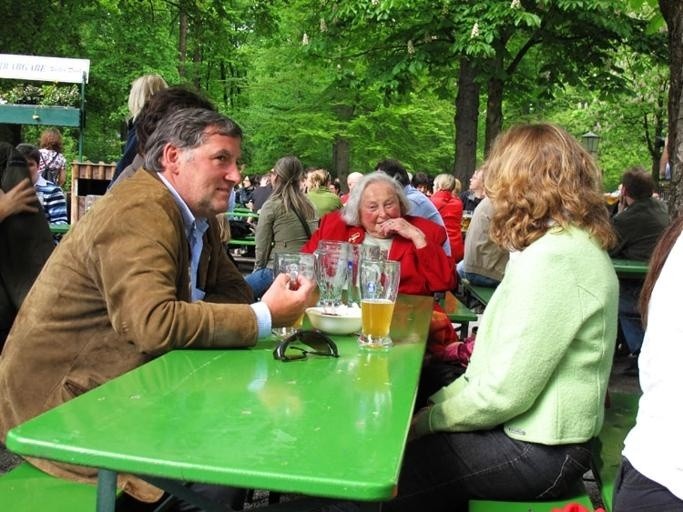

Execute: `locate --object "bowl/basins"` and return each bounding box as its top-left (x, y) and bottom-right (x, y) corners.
top-left (304, 303), bottom-right (361, 336)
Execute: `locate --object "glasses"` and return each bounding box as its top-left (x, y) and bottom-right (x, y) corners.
top-left (274, 330), bottom-right (337, 361)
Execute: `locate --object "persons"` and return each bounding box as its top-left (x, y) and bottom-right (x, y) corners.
top-left (1, 109), bottom-right (319, 512)
top-left (376, 121), bottom-right (622, 512)
top-left (216, 157), bottom-right (508, 408)
top-left (609, 209), bottom-right (683, 511)
top-left (1, 127), bottom-right (68, 354)
top-left (107, 74), bottom-right (169, 192)
top-left (132, 88), bottom-right (220, 150)
top-left (604, 133), bottom-right (671, 372)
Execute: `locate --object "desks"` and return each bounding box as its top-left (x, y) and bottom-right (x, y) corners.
top-left (0, 286), bottom-right (437, 511)
top-left (607, 257), bottom-right (650, 277)
top-left (47, 208), bottom-right (275, 234)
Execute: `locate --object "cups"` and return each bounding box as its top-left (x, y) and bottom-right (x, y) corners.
top-left (358, 258), bottom-right (401, 350)
top-left (311, 239), bottom-right (381, 307)
top-left (270, 251), bottom-right (315, 338)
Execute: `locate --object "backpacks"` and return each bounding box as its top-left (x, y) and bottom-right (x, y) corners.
top-left (39, 152), bottom-right (61, 184)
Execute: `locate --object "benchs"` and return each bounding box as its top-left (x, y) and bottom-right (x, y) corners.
top-left (432, 284), bottom-right (477, 345)
top-left (2, 453), bottom-right (100, 511)
top-left (468, 494), bottom-right (596, 510)
top-left (224, 236), bottom-right (275, 257)
top-left (583, 382), bottom-right (649, 511)
top-left (460, 273), bottom-right (503, 316)
